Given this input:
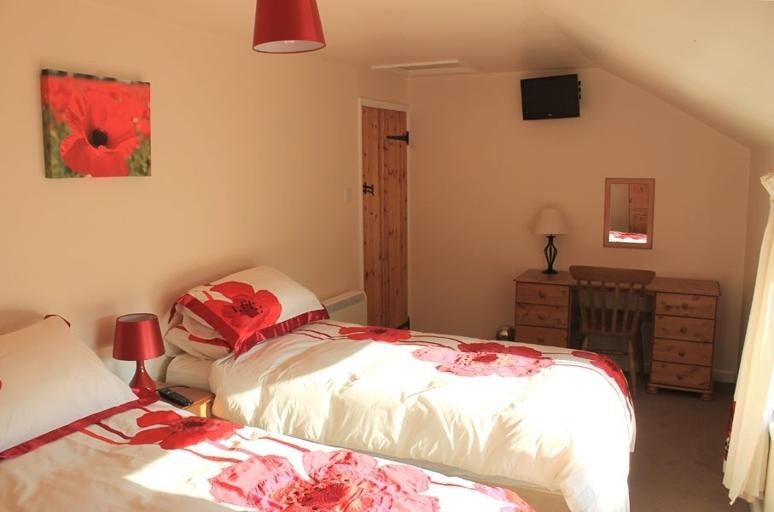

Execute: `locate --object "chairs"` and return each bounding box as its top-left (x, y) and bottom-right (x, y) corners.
top-left (571, 266), bottom-right (653, 399)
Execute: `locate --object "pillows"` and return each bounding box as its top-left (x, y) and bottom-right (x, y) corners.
top-left (168, 265), bottom-right (330, 362)
top-left (1, 316), bottom-right (147, 466)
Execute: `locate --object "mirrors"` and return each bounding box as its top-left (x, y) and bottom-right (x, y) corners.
top-left (603, 177), bottom-right (655, 249)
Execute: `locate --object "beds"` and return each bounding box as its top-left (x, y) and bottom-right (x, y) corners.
top-left (0, 398), bottom-right (533, 511)
top-left (165, 319), bottom-right (637, 512)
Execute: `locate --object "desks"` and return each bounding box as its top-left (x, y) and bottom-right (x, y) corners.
top-left (515, 269), bottom-right (721, 401)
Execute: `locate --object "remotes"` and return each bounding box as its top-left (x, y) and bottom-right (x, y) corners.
top-left (158, 387), bottom-right (192, 407)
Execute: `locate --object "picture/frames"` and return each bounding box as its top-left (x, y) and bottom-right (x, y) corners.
top-left (39, 69), bottom-right (150, 179)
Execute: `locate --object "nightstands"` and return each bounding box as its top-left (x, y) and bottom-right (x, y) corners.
top-left (157, 384), bottom-right (216, 419)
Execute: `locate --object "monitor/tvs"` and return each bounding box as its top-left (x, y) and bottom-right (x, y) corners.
top-left (520, 73), bottom-right (580, 121)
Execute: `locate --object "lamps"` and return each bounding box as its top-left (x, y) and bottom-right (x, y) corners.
top-left (252, 0), bottom-right (326, 55)
top-left (113, 314), bottom-right (166, 395)
top-left (534, 208), bottom-right (568, 275)
